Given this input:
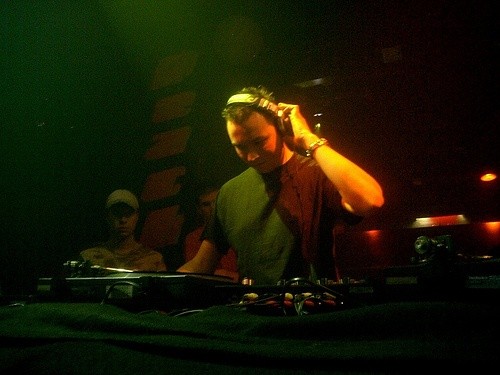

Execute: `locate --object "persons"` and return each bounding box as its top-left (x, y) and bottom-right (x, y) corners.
top-left (185, 182), bottom-right (240, 281)
top-left (76, 189), bottom-right (168, 272)
top-left (176, 87), bottom-right (385, 283)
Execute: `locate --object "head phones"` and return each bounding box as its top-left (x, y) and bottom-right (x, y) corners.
top-left (226, 93), bottom-right (292, 138)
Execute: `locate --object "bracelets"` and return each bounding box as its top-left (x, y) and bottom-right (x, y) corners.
top-left (306, 137), bottom-right (328, 157)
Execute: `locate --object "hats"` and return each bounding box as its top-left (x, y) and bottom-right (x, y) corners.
top-left (105, 190), bottom-right (140, 212)
